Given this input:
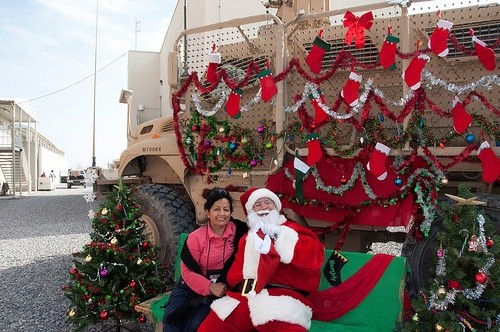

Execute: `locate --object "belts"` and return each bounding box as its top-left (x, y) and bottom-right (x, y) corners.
top-left (230, 276), bottom-right (311, 299)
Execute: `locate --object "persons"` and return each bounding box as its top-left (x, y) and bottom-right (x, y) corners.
top-left (162, 187), bottom-right (325, 332)
top-left (49, 170), bottom-right (56, 192)
top-left (41, 172), bottom-right (46, 177)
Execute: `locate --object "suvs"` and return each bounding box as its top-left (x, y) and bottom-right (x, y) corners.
top-left (67, 169), bottom-right (87, 189)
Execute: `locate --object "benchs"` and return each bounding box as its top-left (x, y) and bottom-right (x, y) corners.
top-left (134, 232), bottom-right (408, 332)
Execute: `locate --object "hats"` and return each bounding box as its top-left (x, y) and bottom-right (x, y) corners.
top-left (240, 187), bottom-right (282, 218)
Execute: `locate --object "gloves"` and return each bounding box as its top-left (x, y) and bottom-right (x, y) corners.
top-left (264, 223), bottom-right (283, 236)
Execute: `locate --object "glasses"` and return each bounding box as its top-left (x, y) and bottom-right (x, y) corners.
top-left (253, 201), bottom-right (273, 207)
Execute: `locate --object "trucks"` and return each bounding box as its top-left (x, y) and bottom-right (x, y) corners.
top-left (115, 0), bottom-right (500, 304)
